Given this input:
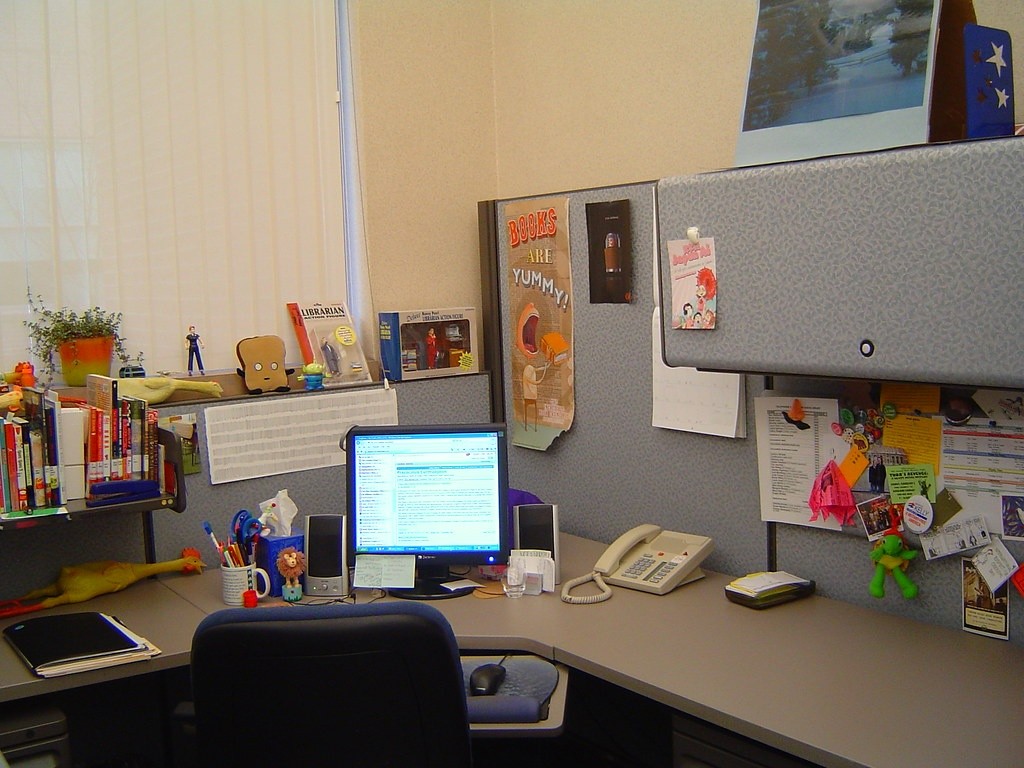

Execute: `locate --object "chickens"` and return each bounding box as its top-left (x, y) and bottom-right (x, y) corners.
top-left (0, 546), bottom-right (209, 620)
top-left (0, 385), bottom-right (25, 412)
top-left (58, 376), bottom-right (223, 406)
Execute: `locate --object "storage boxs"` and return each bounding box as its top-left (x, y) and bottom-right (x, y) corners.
top-left (378, 305), bottom-right (479, 382)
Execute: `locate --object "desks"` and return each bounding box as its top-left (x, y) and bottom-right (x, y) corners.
top-left (154, 529), bottom-right (1024, 768)
top-left (0, 578), bottom-right (208, 704)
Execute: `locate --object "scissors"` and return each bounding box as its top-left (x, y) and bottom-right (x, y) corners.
top-left (231, 509), bottom-right (261, 542)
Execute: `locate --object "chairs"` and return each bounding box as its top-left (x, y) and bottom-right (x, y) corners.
top-left (189, 598), bottom-right (479, 768)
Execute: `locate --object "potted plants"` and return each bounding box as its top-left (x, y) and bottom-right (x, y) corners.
top-left (21, 285), bottom-right (146, 388)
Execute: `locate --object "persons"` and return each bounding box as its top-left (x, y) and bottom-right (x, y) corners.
top-left (863, 499), bottom-right (897, 534)
top-left (426, 327), bottom-right (437, 369)
top-left (185, 326), bottom-right (205, 377)
top-left (977, 578), bottom-right (1005, 613)
top-left (869, 456), bottom-right (886, 492)
top-left (321, 338), bottom-right (343, 377)
top-left (0, 374), bottom-right (176, 514)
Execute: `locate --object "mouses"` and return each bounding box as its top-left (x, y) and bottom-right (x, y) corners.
top-left (469, 663), bottom-right (506, 696)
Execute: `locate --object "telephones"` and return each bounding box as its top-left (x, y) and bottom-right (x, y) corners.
top-left (595, 524), bottom-right (714, 595)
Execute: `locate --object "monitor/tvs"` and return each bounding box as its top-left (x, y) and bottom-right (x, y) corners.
top-left (344, 421), bottom-right (509, 601)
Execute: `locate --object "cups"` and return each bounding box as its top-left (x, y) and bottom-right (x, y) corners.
top-left (501, 569), bottom-right (527, 598)
top-left (220, 562), bottom-right (270, 605)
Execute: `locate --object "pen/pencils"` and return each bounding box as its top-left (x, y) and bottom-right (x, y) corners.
top-left (203, 520), bottom-right (259, 567)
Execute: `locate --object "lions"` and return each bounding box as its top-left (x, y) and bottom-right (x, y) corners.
top-left (276, 546), bottom-right (307, 587)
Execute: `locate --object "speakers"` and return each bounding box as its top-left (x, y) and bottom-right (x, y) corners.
top-left (304, 514), bottom-right (349, 597)
top-left (513, 503), bottom-right (560, 584)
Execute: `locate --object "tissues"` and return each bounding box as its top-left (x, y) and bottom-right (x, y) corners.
top-left (255, 489), bottom-right (305, 596)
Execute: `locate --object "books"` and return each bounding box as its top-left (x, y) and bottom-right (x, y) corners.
top-left (652, 307), bottom-right (747, 438)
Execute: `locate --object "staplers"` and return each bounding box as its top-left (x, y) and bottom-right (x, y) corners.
top-left (85, 479), bottom-right (160, 508)
